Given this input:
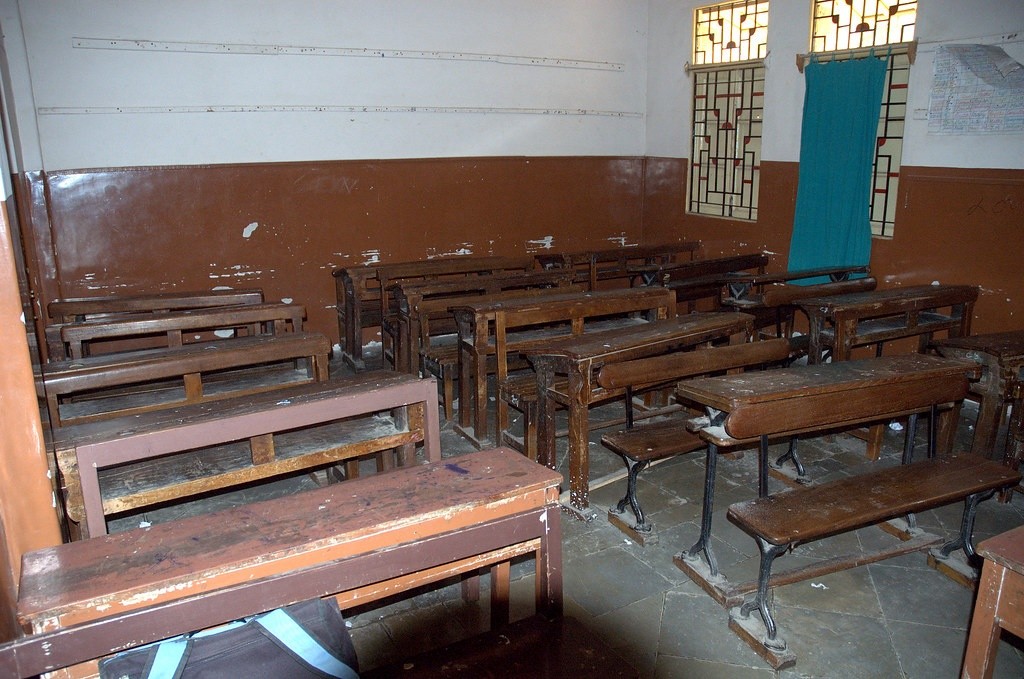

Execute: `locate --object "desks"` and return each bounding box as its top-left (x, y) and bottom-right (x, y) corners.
top-left (384, 268), bottom-right (576, 436)
top-left (16, 447), bottom-right (563, 679)
top-left (53, 370), bottom-right (415, 541)
top-left (929, 329), bottom-right (1024, 462)
top-left (447, 287), bottom-right (676, 452)
top-left (674, 353), bottom-right (983, 604)
top-left (960, 525), bottom-right (1024, 679)
top-left (792, 284), bottom-right (971, 444)
top-left (627, 254), bottom-right (761, 325)
top-left (333, 260), bottom-right (442, 376)
top-left (519, 311), bottom-right (757, 523)
top-left (47, 287), bottom-right (264, 323)
top-left (46, 311), bottom-right (204, 363)
top-left (45, 333), bottom-right (324, 428)
top-left (717, 266), bottom-right (866, 339)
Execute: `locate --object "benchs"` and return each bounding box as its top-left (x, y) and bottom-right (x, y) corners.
top-left (376, 256), bottom-right (1024, 679)
top-left (44, 337), bottom-right (332, 430)
top-left (60, 305), bottom-right (307, 369)
top-left (0, 504), bottom-right (643, 679)
top-left (76, 378), bottom-right (480, 601)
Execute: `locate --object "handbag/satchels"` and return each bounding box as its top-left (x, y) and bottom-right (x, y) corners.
top-left (98, 596), bottom-right (361, 679)
